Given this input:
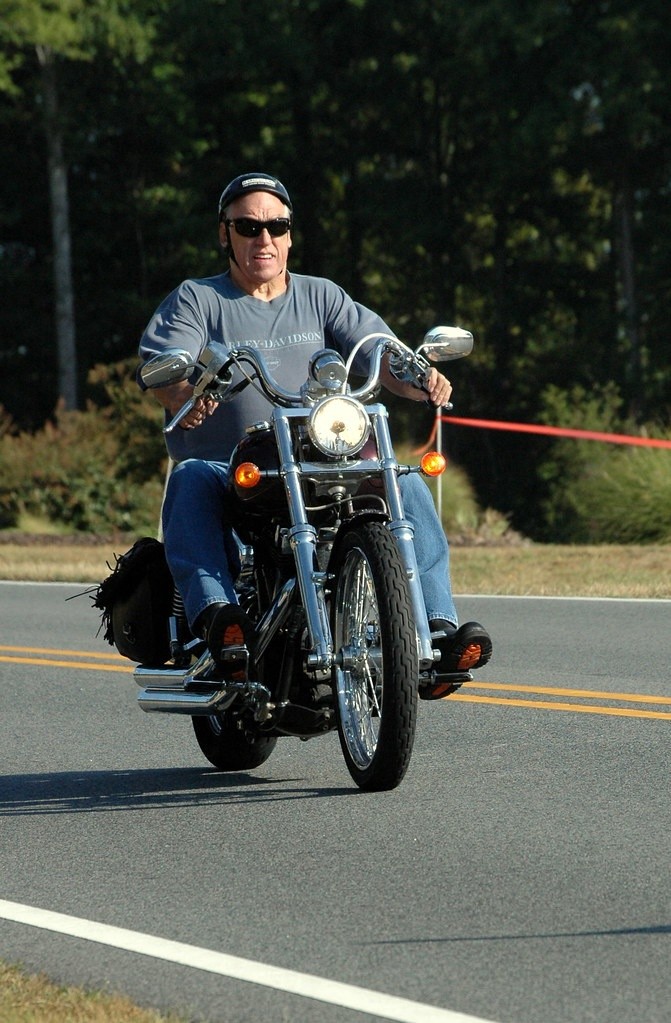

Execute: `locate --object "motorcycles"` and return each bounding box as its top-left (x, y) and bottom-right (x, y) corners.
top-left (132, 326), bottom-right (476, 792)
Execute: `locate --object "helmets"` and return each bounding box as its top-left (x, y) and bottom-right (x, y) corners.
top-left (218, 173), bottom-right (292, 219)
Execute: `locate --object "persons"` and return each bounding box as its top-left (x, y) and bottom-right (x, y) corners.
top-left (138, 173), bottom-right (492, 701)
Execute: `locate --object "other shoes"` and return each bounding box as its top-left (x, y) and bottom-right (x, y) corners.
top-left (205, 601), bottom-right (249, 684)
top-left (418, 619), bottom-right (493, 699)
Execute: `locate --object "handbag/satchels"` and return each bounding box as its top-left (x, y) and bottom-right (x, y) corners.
top-left (63, 537), bottom-right (173, 665)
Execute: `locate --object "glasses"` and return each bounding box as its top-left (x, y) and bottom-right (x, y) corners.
top-left (224, 217), bottom-right (293, 238)
